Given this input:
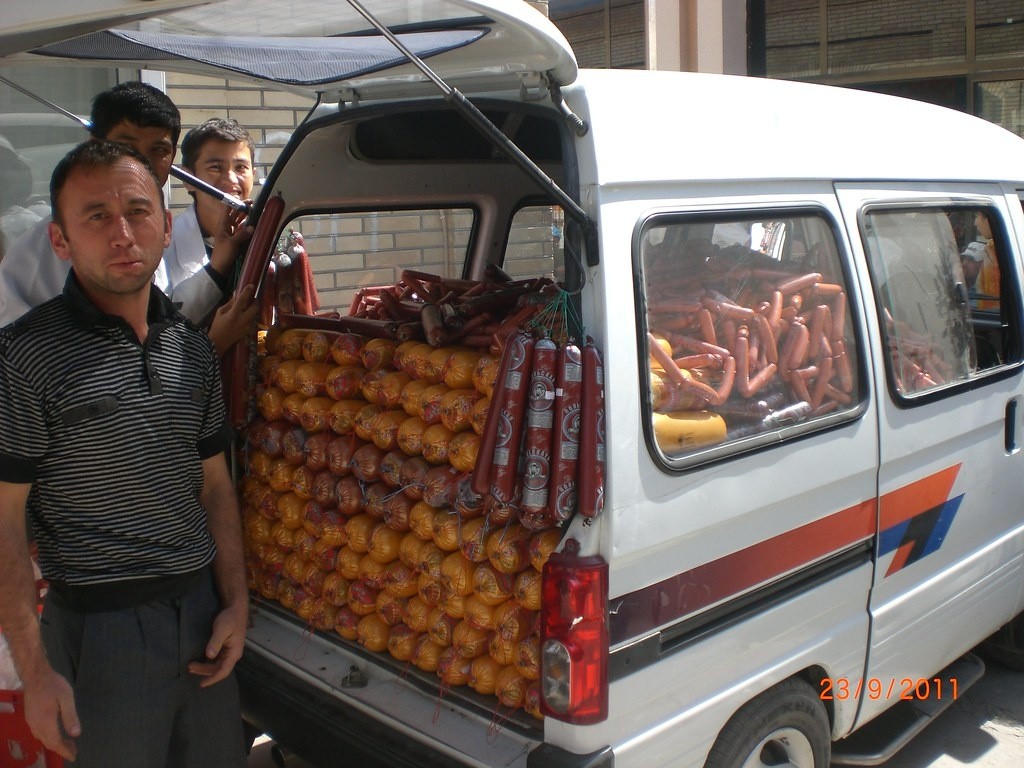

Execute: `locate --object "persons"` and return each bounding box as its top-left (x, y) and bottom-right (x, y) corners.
top-left (865, 236), bottom-right (904, 290)
top-left (0, 135), bottom-right (53, 264)
top-left (973, 207), bottom-right (1002, 311)
top-left (0, 80), bottom-right (255, 768)
top-left (938, 240), bottom-right (987, 299)
top-left (711, 222), bottom-right (752, 250)
top-left (2, 138), bottom-right (250, 768)
top-left (154, 115), bottom-right (277, 362)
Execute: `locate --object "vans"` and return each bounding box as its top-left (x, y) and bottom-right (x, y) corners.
top-left (0, 0), bottom-right (1024, 766)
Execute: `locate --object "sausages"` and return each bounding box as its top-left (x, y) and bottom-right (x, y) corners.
top-left (241, 225), bottom-right (953, 722)
